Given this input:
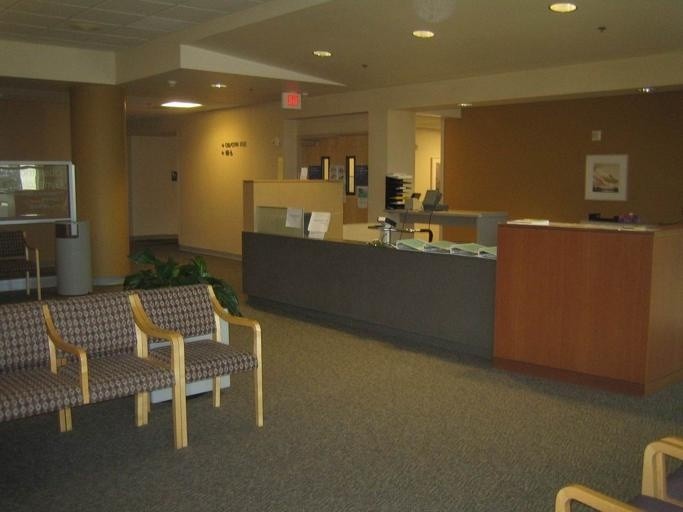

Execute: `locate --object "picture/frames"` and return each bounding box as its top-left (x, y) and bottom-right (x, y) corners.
top-left (583, 153), bottom-right (630, 202)
top-left (430, 157), bottom-right (441, 190)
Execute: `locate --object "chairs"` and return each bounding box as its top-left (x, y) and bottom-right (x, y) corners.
top-left (0, 229), bottom-right (43, 301)
top-left (44, 290), bottom-right (182, 449)
top-left (131, 286), bottom-right (263, 429)
top-left (2, 300), bottom-right (67, 433)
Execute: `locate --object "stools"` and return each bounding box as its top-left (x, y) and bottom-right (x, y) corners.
top-left (554, 441), bottom-right (683, 512)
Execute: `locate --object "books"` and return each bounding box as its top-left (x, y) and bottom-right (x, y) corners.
top-left (507, 218), bottom-right (550, 227)
top-left (395, 238), bottom-right (498, 261)
top-left (385, 173), bottom-right (413, 209)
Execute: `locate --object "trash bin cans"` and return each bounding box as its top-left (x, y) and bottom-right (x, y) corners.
top-left (54, 220), bottom-right (94, 295)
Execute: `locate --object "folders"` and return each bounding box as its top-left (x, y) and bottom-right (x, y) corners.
top-left (396, 239), bottom-right (497, 258)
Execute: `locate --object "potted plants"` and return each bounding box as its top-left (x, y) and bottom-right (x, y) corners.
top-left (120, 247), bottom-right (243, 406)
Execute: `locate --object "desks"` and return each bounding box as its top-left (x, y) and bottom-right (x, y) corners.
top-left (384, 207), bottom-right (508, 246)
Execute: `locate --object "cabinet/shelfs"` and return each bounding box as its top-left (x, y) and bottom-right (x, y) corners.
top-left (242, 179), bottom-right (346, 241)
